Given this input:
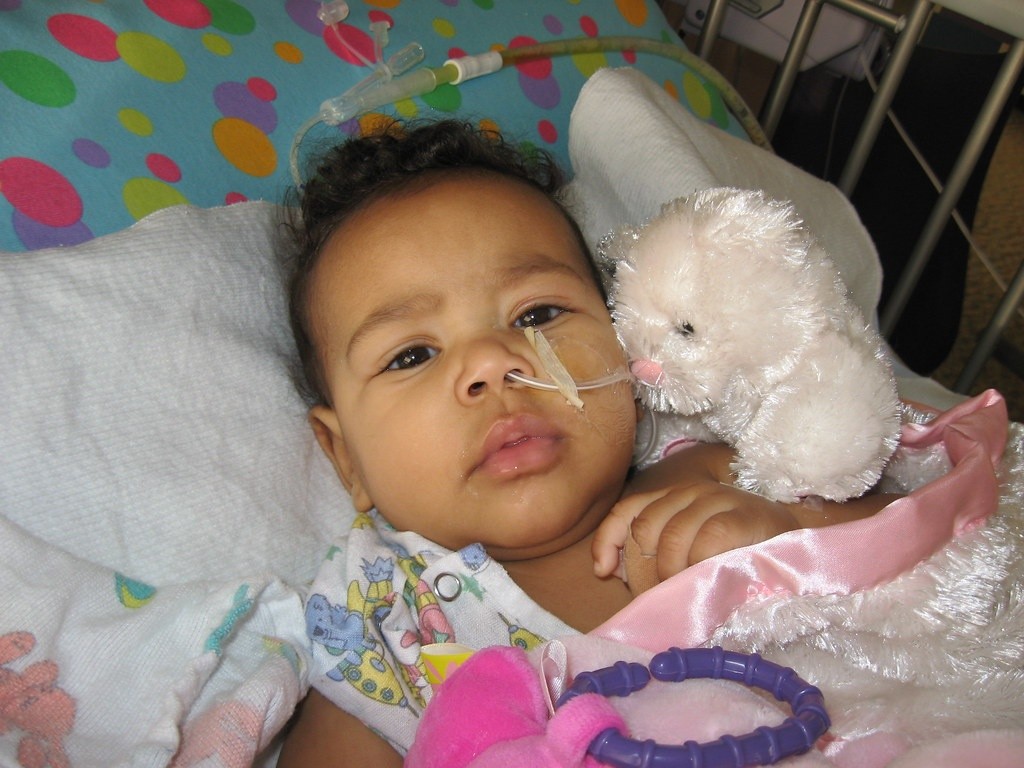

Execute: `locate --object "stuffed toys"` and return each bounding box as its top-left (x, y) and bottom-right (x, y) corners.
top-left (606, 186), bottom-right (902, 500)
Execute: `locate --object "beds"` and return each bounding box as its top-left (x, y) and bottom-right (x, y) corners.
top-left (0, 0), bottom-right (1024, 768)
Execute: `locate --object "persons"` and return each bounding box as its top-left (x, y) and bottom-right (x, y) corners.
top-left (276, 118), bottom-right (917, 767)
